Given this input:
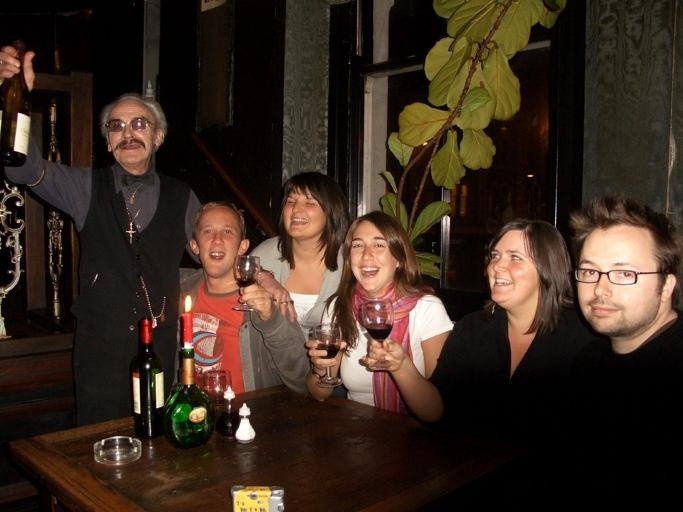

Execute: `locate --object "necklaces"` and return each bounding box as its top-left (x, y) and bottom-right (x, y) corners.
top-left (127, 180), bottom-right (146, 204)
top-left (120, 202), bottom-right (142, 243)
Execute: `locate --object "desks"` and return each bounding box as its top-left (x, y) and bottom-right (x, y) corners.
top-left (8, 384), bottom-right (486, 512)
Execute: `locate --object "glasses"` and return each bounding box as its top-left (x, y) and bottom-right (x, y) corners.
top-left (105, 118), bottom-right (153, 132)
top-left (574, 268), bottom-right (659, 286)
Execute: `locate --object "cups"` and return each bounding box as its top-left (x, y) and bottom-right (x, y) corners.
top-left (178, 368), bottom-right (203, 393)
top-left (203, 370), bottom-right (232, 434)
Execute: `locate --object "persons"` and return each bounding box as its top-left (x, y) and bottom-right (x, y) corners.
top-left (0, 45), bottom-right (298, 423)
top-left (248, 170), bottom-right (356, 396)
top-left (548, 193), bottom-right (682, 511)
top-left (182, 202), bottom-right (316, 394)
top-left (358, 218), bottom-right (590, 512)
top-left (305, 211), bottom-right (454, 416)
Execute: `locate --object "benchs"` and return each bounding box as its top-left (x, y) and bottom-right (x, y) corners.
top-left (0, 332), bottom-right (76, 505)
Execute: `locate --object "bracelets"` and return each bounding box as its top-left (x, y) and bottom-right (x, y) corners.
top-left (257, 270), bottom-right (274, 285)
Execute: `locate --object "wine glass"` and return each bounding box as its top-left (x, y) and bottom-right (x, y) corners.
top-left (232, 254), bottom-right (262, 311)
top-left (361, 296), bottom-right (394, 370)
top-left (313, 324), bottom-right (344, 388)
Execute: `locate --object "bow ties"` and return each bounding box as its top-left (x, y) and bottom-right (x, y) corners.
top-left (124, 174), bottom-right (154, 187)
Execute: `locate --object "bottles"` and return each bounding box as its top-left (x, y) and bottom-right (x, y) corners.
top-left (235, 402), bottom-right (257, 444)
top-left (1, 40), bottom-right (32, 166)
top-left (215, 384), bottom-right (239, 441)
top-left (163, 348), bottom-right (214, 448)
top-left (129, 318), bottom-right (165, 439)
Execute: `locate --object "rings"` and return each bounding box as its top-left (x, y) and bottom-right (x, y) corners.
top-left (289, 298), bottom-right (295, 305)
top-left (273, 298), bottom-right (281, 302)
top-left (283, 300), bottom-right (289, 306)
